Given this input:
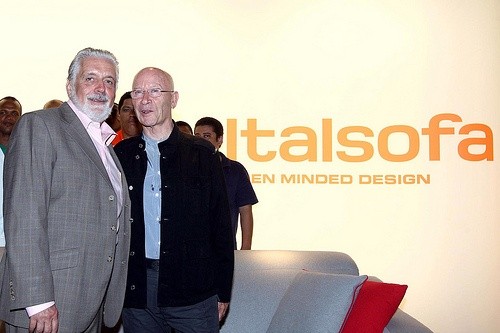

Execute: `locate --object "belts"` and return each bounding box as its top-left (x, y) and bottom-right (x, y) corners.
top-left (146, 258), bottom-right (160, 272)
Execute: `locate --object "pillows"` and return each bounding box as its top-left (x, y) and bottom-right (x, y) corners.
top-left (342, 281), bottom-right (408, 333)
top-left (266, 268), bottom-right (368, 333)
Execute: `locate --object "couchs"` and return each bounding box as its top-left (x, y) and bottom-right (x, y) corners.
top-left (218, 250), bottom-right (435, 333)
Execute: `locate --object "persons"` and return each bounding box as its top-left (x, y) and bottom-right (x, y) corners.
top-left (43, 91), bottom-right (193, 148)
top-left (113, 67), bottom-right (235, 333)
top-left (0, 97), bottom-right (23, 264)
top-left (0, 47), bottom-right (131, 333)
top-left (194, 117), bottom-right (260, 250)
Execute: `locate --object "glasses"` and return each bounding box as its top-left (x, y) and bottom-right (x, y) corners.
top-left (130, 88), bottom-right (174, 99)
